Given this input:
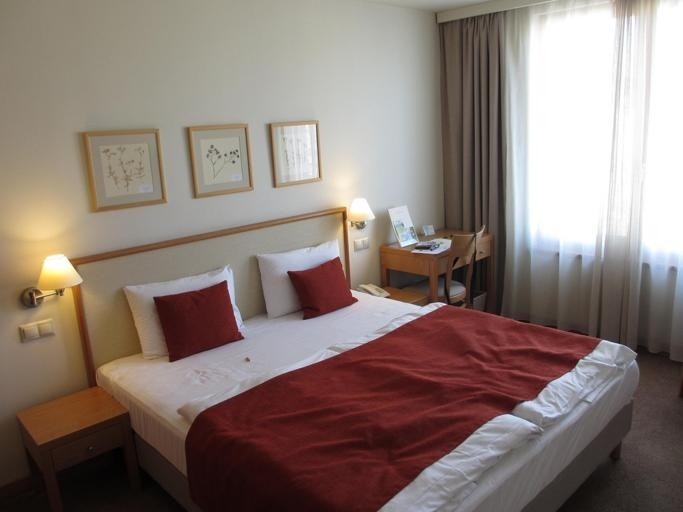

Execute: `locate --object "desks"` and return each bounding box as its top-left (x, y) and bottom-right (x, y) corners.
top-left (379, 228), bottom-right (495, 313)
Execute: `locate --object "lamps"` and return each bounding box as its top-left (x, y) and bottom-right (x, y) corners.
top-left (346, 198), bottom-right (375, 229)
top-left (21, 254), bottom-right (84, 309)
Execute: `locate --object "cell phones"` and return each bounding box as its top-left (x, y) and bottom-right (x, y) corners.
top-left (416, 242), bottom-right (436, 248)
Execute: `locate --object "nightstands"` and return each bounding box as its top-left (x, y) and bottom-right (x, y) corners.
top-left (383, 287), bottom-right (428, 307)
top-left (15, 385), bottom-right (140, 512)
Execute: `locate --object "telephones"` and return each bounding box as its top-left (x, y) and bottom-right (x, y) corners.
top-left (356, 284), bottom-right (391, 297)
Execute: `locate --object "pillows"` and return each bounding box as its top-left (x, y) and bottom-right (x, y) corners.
top-left (123, 261), bottom-right (247, 363)
top-left (255, 238), bottom-right (358, 322)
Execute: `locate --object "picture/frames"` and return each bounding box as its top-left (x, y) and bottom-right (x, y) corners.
top-left (268, 120), bottom-right (323, 188)
top-left (83, 128), bottom-right (168, 212)
top-left (188, 123), bottom-right (255, 198)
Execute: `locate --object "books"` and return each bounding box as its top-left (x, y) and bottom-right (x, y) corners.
top-left (386, 204), bottom-right (419, 248)
top-left (411, 238), bottom-right (452, 255)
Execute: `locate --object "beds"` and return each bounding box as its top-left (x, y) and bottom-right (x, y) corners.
top-left (69, 207), bottom-right (641, 512)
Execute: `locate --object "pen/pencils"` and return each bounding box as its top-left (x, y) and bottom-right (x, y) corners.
top-left (431, 247), bottom-right (436, 251)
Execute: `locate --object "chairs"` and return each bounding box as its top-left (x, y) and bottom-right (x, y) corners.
top-left (401, 223), bottom-right (486, 309)
